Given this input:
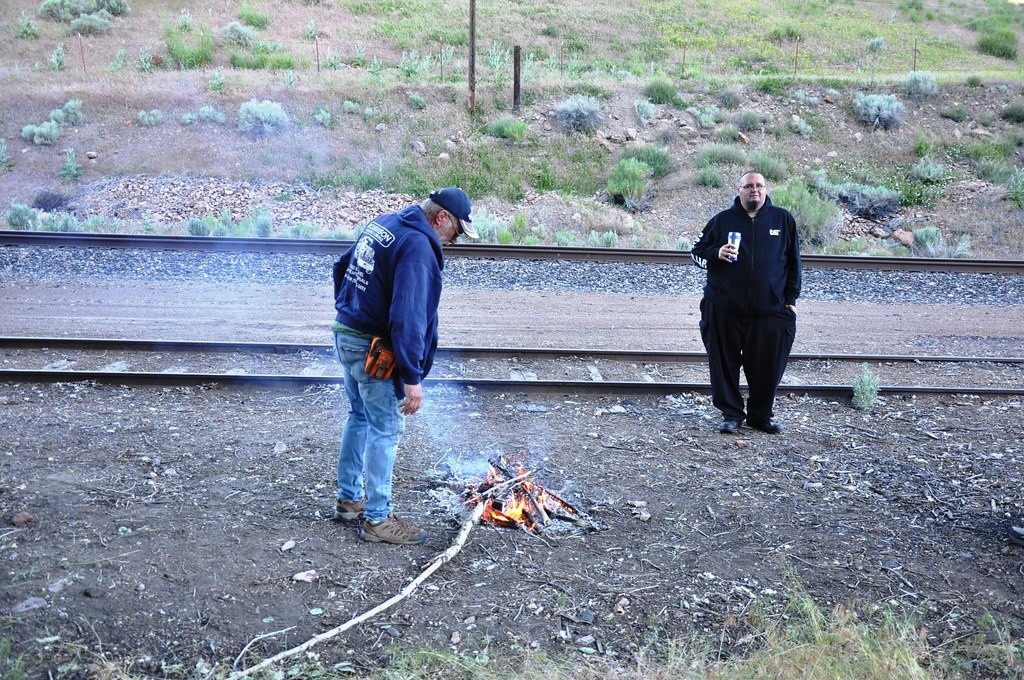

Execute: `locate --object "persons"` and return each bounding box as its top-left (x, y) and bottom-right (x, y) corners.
top-left (691, 170), bottom-right (802, 434)
top-left (330, 187), bottom-right (480, 545)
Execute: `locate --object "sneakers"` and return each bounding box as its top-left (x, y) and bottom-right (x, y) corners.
top-left (334, 493), bottom-right (368, 521)
top-left (361, 512), bottom-right (427, 544)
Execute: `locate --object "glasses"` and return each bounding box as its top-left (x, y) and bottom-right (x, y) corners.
top-left (739, 183), bottom-right (766, 191)
top-left (437, 209), bottom-right (460, 240)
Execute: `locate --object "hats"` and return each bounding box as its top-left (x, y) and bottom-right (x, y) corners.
top-left (429, 187), bottom-right (479, 238)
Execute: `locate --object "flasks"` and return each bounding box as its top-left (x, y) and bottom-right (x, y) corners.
top-left (728, 232), bottom-right (741, 262)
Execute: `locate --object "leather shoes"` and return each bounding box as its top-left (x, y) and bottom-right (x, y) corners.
top-left (755, 421), bottom-right (784, 434)
top-left (717, 420), bottom-right (740, 433)
